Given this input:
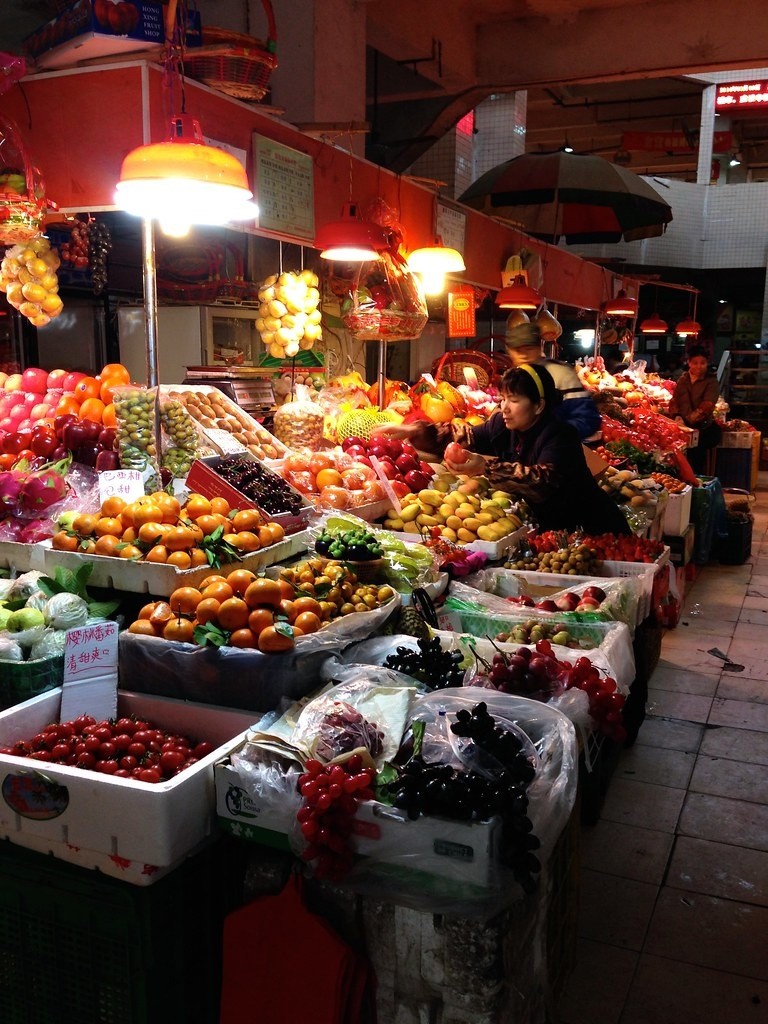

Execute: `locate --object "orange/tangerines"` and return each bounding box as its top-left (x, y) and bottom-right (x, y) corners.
top-left (52, 492), bottom-right (324, 652)
top-left (598, 412), bottom-right (683, 454)
top-left (55, 364), bottom-right (143, 427)
top-left (650, 471), bottom-right (688, 493)
top-left (281, 450), bottom-right (383, 509)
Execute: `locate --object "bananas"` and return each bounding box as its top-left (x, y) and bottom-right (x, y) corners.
top-left (602, 328), bottom-right (632, 344)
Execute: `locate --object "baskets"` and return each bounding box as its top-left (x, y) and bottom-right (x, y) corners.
top-left (338, 253), bottom-right (429, 341)
top-left (494, 558), bottom-right (658, 628)
top-left (0, 117), bottom-right (46, 244)
top-left (155, 237), bottom-right (263, 305)
top-left (432, 349), bottom-right (497, 393)
top-left (716, 431), bottom-right (753, 449)
top-left (161, 0), bottom-right (276, 102)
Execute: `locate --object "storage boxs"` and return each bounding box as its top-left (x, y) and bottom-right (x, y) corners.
top-left (0, 378), bottom-right (692, 1023)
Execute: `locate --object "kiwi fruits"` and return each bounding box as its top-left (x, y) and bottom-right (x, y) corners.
top-left (170, 390), bottom-right (286, 460)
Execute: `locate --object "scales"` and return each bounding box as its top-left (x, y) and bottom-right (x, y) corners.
top-left (181, 365), bottom-right (279, 410)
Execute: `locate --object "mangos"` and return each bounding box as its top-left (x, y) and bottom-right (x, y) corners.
top-left (380, 472), bottom-right (523, 547)
top-left (596, 466), bottom-right (654, 504)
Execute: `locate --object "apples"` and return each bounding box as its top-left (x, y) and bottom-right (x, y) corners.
top-left (343, 434), bottom-right (467, 495)
top-left (505, 587), bottom-right (607, 615)
top-left (0, 369), bottom-right (119, 477)
top-left (366, 284), bottom-right (419, 314)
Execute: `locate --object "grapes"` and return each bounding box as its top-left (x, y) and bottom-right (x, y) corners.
top-left (716, 419), bottom-right (751, 433)
top-left (63, 220), bottom-right (113, 295)
top-left (291, 635), bottom-right (628, 885)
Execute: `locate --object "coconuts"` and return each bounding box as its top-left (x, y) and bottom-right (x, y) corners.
top-left (507, 309), bottom-right (562, 339)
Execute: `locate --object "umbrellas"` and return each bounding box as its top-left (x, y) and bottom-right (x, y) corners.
top-left (457, 150), bottom-right (674, 248)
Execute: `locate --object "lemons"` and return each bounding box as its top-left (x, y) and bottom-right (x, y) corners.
top-left (255, 271), bottom-right (321, 360)
top-left (0, 237), bottom-right (64, 328)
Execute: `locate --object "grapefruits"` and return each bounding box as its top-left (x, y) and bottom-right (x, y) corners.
top-left (285, 372), bottom-right (484, 441)
top-left (575, 365), bottom-right (644, 404)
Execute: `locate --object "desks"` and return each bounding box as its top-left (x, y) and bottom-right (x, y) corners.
top-left (710, 429), bottom-right (760, 493)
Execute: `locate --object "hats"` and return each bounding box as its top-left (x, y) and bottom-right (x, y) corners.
top-left (504, 324), bottom-right (543, 348)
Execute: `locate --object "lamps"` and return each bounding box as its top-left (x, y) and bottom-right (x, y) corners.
top-left (675, 316), bottom-right (699, 338)
top-left (605, 288), bottom-right (637, 316)
top-left (114, 35), bottom-right (255, 240)
top-left (319, 131), bottom-right (381, 264)
top-left (407, 182), bottom-right (467, 292)
top-left (494, 219), bottom-right (542, 311)
top-left (640, 313), bottom-right (667, 334)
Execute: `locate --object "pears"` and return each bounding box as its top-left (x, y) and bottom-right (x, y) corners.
top-left (1, 569), bottom-right (89, 663)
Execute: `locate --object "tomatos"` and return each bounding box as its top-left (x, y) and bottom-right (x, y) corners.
top-left (0, 713), bottom-right (213, 785)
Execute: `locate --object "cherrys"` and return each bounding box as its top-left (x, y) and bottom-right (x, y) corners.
top-left (212, 459), bottom-right (303, 517)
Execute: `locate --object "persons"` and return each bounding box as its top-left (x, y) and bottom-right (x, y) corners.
top-left (668, 346), bottom-right (721, 474)
top-left (369, 363), bottom-right (634, 539)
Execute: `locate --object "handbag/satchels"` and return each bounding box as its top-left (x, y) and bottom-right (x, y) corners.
top-left (697, 420), bottom-right (723, 449)
